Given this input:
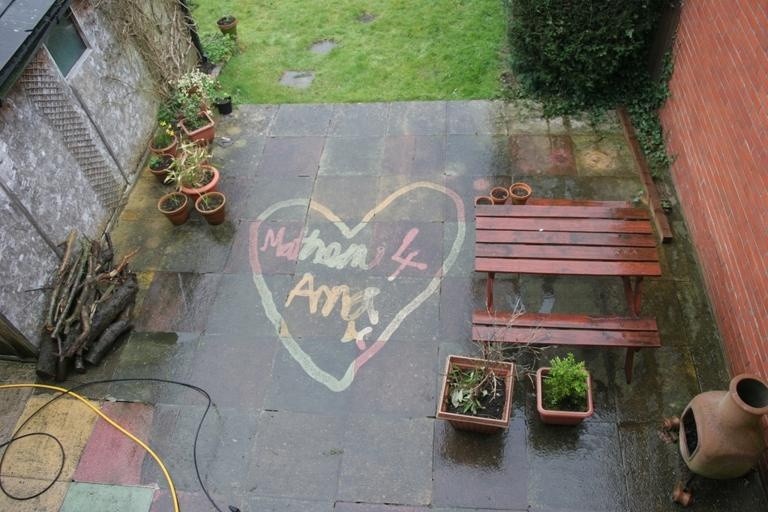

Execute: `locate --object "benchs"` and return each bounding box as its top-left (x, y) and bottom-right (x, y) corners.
top-left (470, 307), bottom-right (664, 389)
top-left (502, 197), bottom-right (635, 209)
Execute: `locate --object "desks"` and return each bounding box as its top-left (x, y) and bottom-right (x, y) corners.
top-left (472, 203), bottom-right (662, 315)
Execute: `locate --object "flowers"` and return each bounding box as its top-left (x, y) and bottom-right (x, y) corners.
top-left (150, 116), bottom-right (176, 147)
top-left (167, 69), bottom-right (226, 128)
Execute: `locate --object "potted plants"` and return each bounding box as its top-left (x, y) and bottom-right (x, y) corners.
top-left (147, 149), bottom-right (230, 229)
top-left (534, 349), bottom-right (594, 425)
top-left (217, 16), bottom-right (238, 35)
top-left (213, 91), bottom-right (233, 116)
top-left (436, 287), bottom-right (558, 449)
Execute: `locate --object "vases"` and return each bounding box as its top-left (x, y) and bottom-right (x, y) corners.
top-left (147, 133), bottom-right (180, 160)
top-left (180, 112), bottom-right (216, 144)
top-left (472, 182), bottom-right (531, 208)
